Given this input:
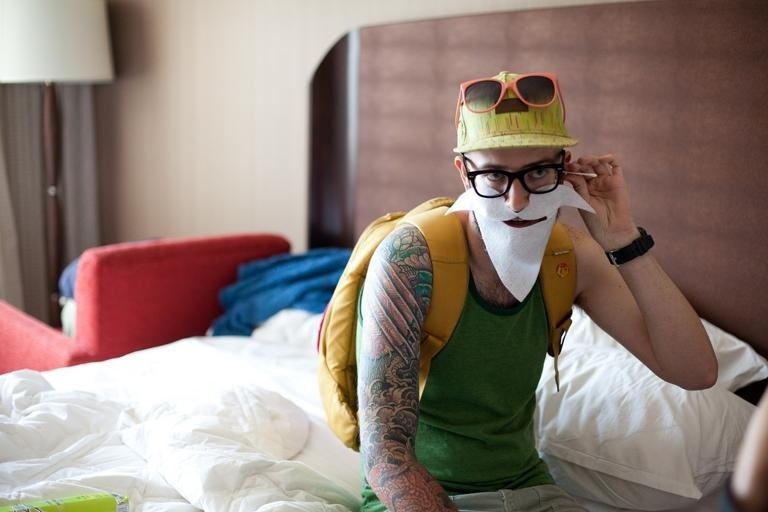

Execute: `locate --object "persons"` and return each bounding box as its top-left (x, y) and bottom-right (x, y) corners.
top-left (357, 71), bottom-right (719, 512)
top-left (730, 386), bottom-right (768, 511)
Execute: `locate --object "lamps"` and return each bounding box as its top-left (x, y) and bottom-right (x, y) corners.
top-left (1, 2), bottom-right (114, 325)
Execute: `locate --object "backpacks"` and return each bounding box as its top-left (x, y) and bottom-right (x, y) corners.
top-left (319, 198), bottom-right (576, 450)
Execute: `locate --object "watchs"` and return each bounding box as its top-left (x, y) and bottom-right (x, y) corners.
top-left (602, 225), bottom-right (654, 269)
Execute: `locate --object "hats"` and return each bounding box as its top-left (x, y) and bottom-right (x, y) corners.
top-left (454, 71), bottom-right (577, 151)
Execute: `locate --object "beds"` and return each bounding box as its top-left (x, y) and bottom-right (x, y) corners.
top-left (1, 341), bottom-right (766, 511)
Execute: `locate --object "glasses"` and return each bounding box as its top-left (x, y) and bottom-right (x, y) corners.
top-left (462, 152), bottom-right (566, 198)
top-left (454, 73), bottom-right (566, 133)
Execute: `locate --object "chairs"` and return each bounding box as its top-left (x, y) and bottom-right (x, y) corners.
top-left (3, 235), bottom-right (293, 369)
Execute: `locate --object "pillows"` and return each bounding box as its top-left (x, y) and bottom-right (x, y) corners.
top-left (257, 292), bottom-right (767, 500)
